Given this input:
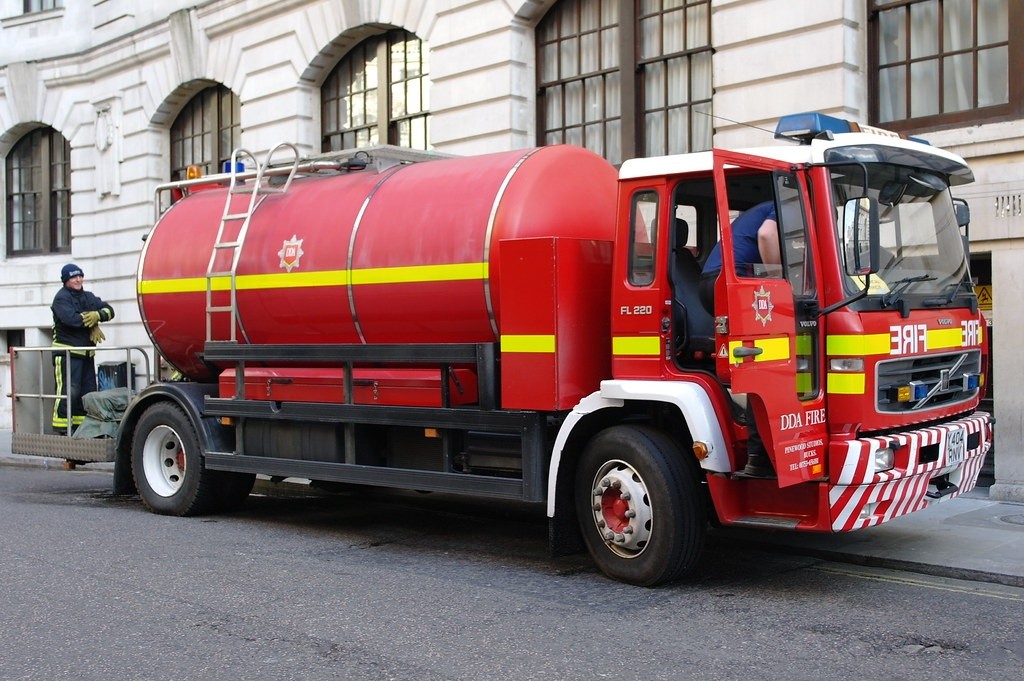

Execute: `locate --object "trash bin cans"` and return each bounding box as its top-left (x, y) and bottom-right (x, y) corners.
top-left (98, 360), bottom-right (136, 392)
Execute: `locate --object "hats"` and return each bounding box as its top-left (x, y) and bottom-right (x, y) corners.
top-left (61, 264), bottom-right (85, 280)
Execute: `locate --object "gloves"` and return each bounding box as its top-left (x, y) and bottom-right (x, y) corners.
top-left (82, 310), bottom-right (101, 326)
top-left (91, 328), bottom-right (105, 346)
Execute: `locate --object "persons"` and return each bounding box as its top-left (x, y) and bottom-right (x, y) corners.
top-left (51, 264), bottom-right (114, 466)
top-left (699, 201), bottom-right (805, 477)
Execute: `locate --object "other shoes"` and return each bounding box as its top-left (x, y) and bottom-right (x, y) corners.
top-left (745, 453), bottom-right (776, 480)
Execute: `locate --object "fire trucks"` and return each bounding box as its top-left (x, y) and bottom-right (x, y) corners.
top-left (114, 111), bottom-right (994, 587)
